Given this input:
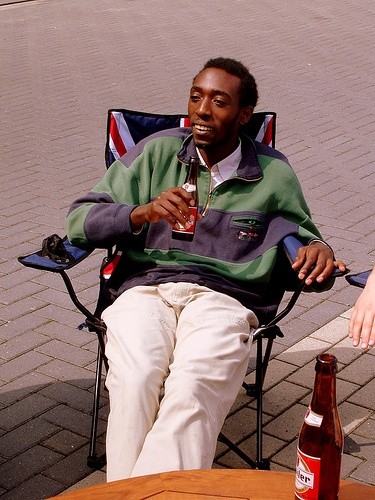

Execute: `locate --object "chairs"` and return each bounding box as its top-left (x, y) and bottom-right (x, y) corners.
top-left (344, 270), bottom-right (373, 288)
top-left (18, 108), bottom-right (352, 470)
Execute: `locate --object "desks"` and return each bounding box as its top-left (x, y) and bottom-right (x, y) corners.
top-left (42, 469), bottom-right (375, 499)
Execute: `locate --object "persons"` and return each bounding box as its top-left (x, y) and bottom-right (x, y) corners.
top-left (66, 57), bottom-right (347, 483)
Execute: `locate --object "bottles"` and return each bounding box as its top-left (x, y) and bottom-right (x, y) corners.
top-left (171, 156), bottom-right (201, 242)
top-left (293, 353), bottom-right (344, 500)
